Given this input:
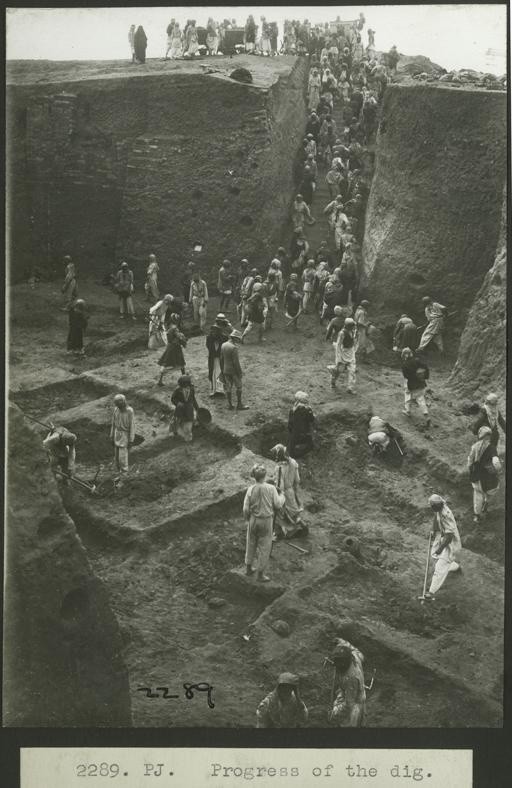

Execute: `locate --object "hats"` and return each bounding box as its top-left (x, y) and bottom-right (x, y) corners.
top-left (279, 673), bottom-right (299, 686)
top-left (216, 314), bottom-right (225, 321)
top-left (171, 313), bottom-right (179, 323)
top-left (250, 465), bottom-right (266, 478)
top-left (230, 330), bottom-right (242, 340)
top-left (330, 646), bottom-right (351, 658)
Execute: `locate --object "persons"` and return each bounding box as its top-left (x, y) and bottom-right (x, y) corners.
top-left (256, 671), bottom-right (309, 730)
top-left (324, 637), bottom-right (366, 731)
